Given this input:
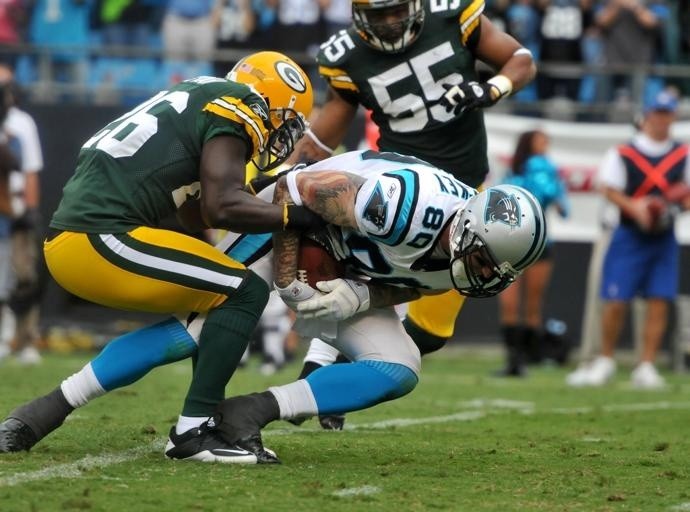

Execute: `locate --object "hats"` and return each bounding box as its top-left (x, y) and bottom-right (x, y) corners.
top-left (644, 90), bottom-right (679, 113)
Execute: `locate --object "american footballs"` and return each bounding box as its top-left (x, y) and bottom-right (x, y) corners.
top-left (297, 236), bottom-right (338, 294)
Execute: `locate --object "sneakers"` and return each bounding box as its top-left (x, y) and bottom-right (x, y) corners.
top-left (0, 401), bottom-right (46, 457)
top-left (565, 353), bottom-right (618, 388)
top-left (163, 415), bottom-right (280, 467)
top-left (628, 357), bottom-right (666, 391)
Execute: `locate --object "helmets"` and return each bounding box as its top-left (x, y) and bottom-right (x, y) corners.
top-left (225, 50), bottom-right (314, 172)
top-left (447, 182), bottom-right (547, 298)
top-left (349, 0), bottom-right (427, 54)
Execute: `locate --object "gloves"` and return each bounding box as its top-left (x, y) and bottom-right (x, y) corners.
top-left (279, 202), bottom-right (330, 237)
top-left (272, 277), bottom-right (331, 317)
top-left (298, 278), bottom-right (371, 322)
top-left (440, 79), bottom-right (505, 118)
